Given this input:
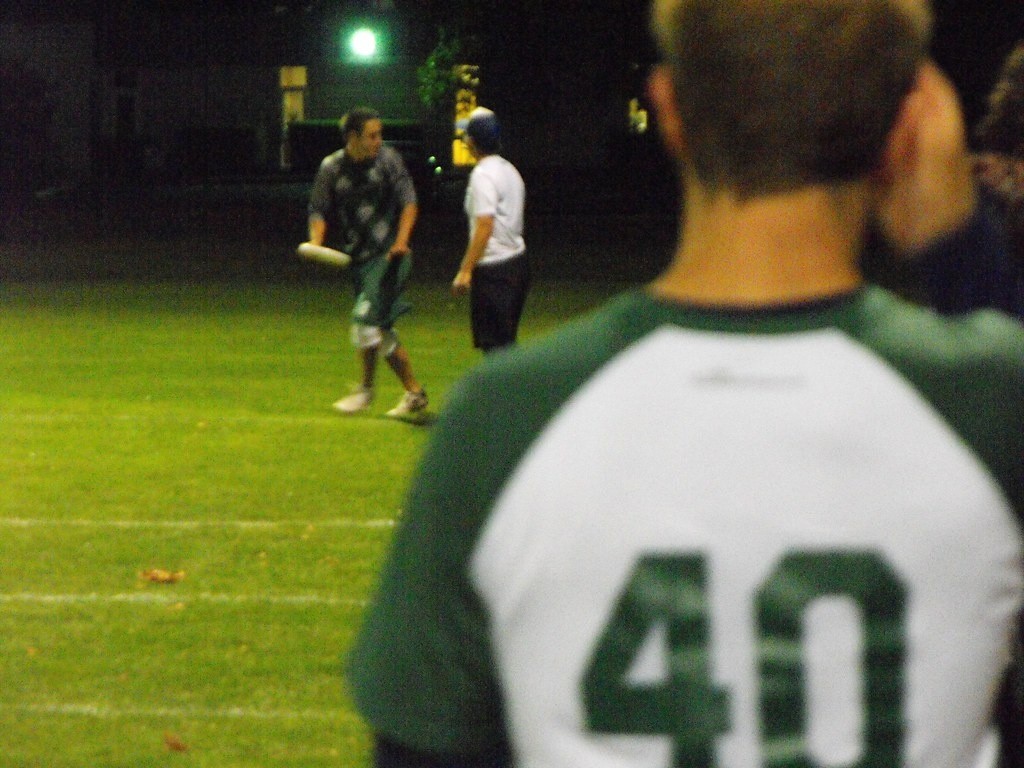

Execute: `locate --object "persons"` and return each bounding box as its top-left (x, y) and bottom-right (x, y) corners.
top-left (451, 107), bottom-right (531, 351)
top-left (307, 105), bottom-right (428, 418)
top-left (348, 1), bottom-right (1024, 768)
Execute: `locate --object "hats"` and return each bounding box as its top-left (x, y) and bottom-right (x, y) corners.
top-left (454, 106), bottom-right (501, 139)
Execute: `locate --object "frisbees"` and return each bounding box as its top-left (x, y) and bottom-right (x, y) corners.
top-left (298, 242), bottom-right (348, 267)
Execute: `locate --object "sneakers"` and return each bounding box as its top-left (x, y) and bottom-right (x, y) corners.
top-left (331, 393), bottom-right (372, 412)
top-left (386, 388), bottom-right (429, 416)
